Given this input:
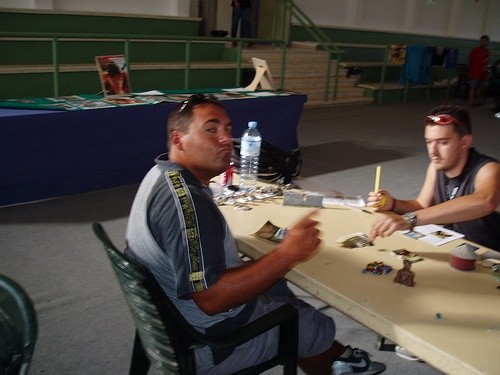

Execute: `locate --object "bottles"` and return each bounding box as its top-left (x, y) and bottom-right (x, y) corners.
top-left (239, 121), bottom-right (262, 185)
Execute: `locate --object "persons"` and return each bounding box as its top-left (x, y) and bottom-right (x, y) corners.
top-left (368, 104), bottom-right (500, 362)
top-left (126, 93), bottom-right (387, 375)
top-left (467, 35), bottom-right (491, 105)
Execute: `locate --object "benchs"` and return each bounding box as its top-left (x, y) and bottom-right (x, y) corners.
top-left (291, 23), bottom-right (500, 102)
top-left (0, 7), bottom-right (257, 99)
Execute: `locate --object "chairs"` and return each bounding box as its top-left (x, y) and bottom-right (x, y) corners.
top-left (92, 221), bottom-right (299, 375)
top-left (0, 274), bottom-right (39, 375)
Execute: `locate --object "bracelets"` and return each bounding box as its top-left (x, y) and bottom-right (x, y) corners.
top-left (390, 199), bottom-right (396, 211)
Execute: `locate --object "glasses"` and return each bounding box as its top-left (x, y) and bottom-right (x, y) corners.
top-left (425, 114), bottom-right (462, 130)
top-left (178, 93), bottom-right (218, 115)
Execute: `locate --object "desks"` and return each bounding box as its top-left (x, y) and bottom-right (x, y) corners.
top-left (210, 171), bottom-right (500, 375)
top-left (0, 89), bottom-right (307, 206)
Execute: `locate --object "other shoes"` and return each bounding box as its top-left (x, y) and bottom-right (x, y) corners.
top-left (395, 345), bottom-right (420, 362)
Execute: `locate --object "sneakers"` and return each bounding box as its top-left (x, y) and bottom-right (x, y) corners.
top-left (331, 345), bottom-right (387, 375)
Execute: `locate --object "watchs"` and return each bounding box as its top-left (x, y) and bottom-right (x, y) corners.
top-left (404, 212), bottom-right (417, 224)
